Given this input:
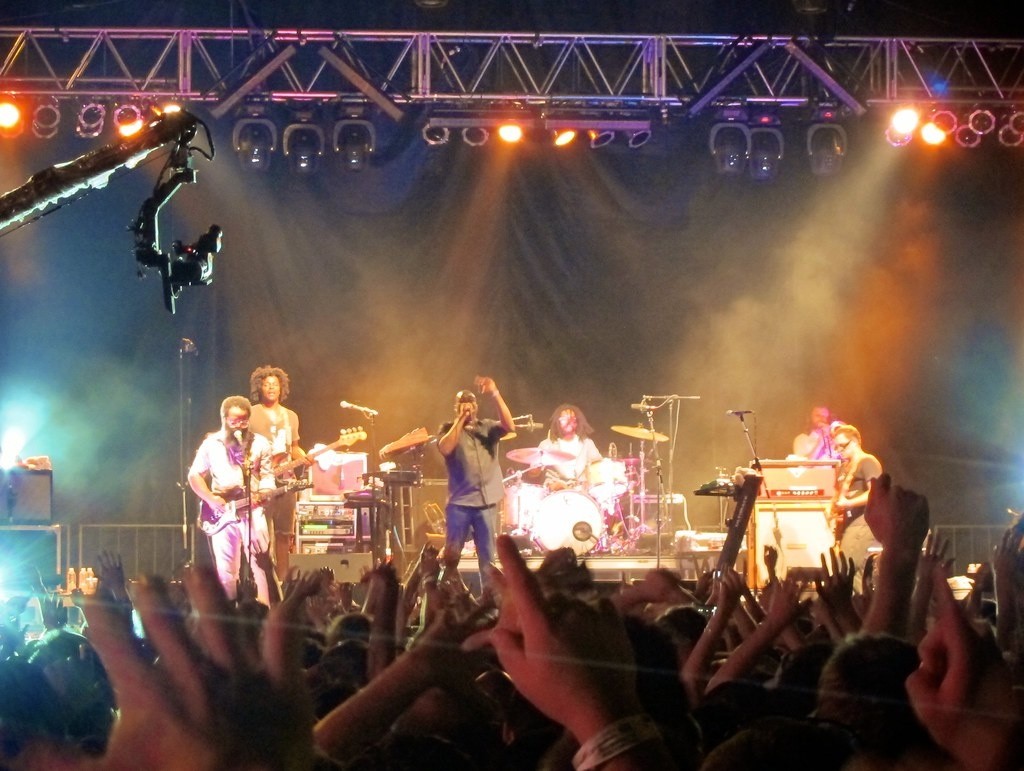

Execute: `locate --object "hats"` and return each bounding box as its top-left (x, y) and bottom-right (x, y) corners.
top-left (455, 390), bottom-right (476, 404)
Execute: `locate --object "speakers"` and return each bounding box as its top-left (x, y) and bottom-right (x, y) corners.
top-left (747, 502), bottom-right (837, 589)
top-left (288, 552), bottom-right (374, 584)
top-left (411, 478), bottom-right (450, 549)
top-left (0, 468), bottom-right (53, 525)
top-left (308, 454), bottom-right (367, 502)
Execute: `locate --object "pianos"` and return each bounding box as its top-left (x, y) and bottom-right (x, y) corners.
top-left (362, 425), bottom-right (430, 549)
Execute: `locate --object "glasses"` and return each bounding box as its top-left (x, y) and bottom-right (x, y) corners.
top-left (834, 438), bottom-right (852, 450)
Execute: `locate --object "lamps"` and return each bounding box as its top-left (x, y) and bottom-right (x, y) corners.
top-left (710, 107), bottom-right (848, 181)
top-left (232, 102), bottom-right (376, 175)
top-left (421, 109), bottom-right (651, 148)
top-left (0, 94), bottom-right (181, 139)
top-left (885, 103), bottom-right (1023, 148)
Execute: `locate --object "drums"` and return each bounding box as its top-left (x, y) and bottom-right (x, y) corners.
top-left (632, 493), bottom-right (684, 536)
top-left (585, 458), bottom-right (628, 500)
top-left (503, 482), bottom-right (545, 529)
top-left (531, 488), bottom-right (605, 557)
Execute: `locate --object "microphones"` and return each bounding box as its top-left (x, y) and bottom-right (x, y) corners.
top-left (726, 410), bottom-right (752, 415)
top-left (234, 430), bottom-right (243, 445)
top-left (340, 401), bottom-right (379, 416)
top-left (632, 403), bottom-right (658, 412)
top-left (515, 423), bottom-right (544, 430)
top-left (183, 338), bottom-right (199, 355)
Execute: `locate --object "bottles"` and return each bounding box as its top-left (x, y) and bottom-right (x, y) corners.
top-left (967, 563), bottom-right (982, 573)
top-left (78, 567), bottom-right (88, 594)
top-left (87, 568), bottom-right (95, 577)
top-left (67, 568), bottom-right (76, 594)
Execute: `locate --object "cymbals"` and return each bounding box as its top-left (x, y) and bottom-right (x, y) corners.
top-left (618, 457), bottom-right (647, 465)
top-left (506, 447), bottom-right (577, 466)
top-left (610, 425), bottom-right (670, 442)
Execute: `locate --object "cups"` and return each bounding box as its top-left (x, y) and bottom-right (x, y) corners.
top-left (85, 578), bottom-right (98, 596)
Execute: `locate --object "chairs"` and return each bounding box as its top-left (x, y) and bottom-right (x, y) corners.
top-left (622, 459), bottom-right (691, 530)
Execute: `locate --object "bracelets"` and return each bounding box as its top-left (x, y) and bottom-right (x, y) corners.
top-left (571, 714), bottom-right (662, 771)
top-left (492, 390), bottom-right (499, 398)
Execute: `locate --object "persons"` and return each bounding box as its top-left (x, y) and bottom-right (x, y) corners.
top-left (437, 375), bottom-right (516, 568)
top-left (833, 426), bottom-right (882, 571)
top-left (188, 397), bottom-right (284, 609)
top-left (792, 404), bottom-right (846, 462)
top-left (248, 365), bottom-right (314, 583)
top-left (529, 404), bottom-right (602, 487)
top-left (0, 473), bottom-right (1024, 771)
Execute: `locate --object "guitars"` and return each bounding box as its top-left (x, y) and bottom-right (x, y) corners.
top-left (832, 473), bottom-right (849, 541)
top-left (199, 477), bottom-right (308, 536)
top-left (270, 425), bottom-right (368, 485)
